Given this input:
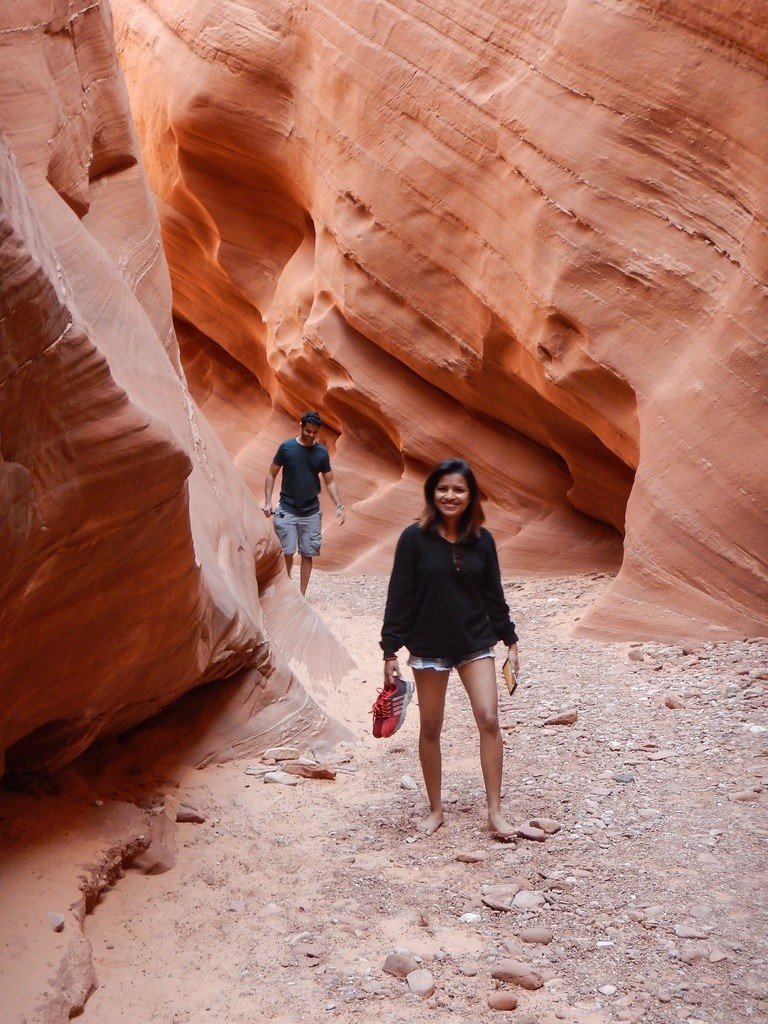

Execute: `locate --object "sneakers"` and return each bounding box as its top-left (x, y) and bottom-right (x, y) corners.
top-left (368, 675), bottom-right (414, 738)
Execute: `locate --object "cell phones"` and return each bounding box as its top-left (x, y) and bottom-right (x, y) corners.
top-left (502, 657), bottom-right (519, 696)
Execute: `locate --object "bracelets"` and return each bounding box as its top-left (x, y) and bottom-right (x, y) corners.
top-left (336, 503), bottom-right (344, 509)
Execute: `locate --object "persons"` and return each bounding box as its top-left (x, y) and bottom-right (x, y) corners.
top-left (379, 457), bottom-right (519, 838)
top-left (262, 410), bottom-right (346, 596)
